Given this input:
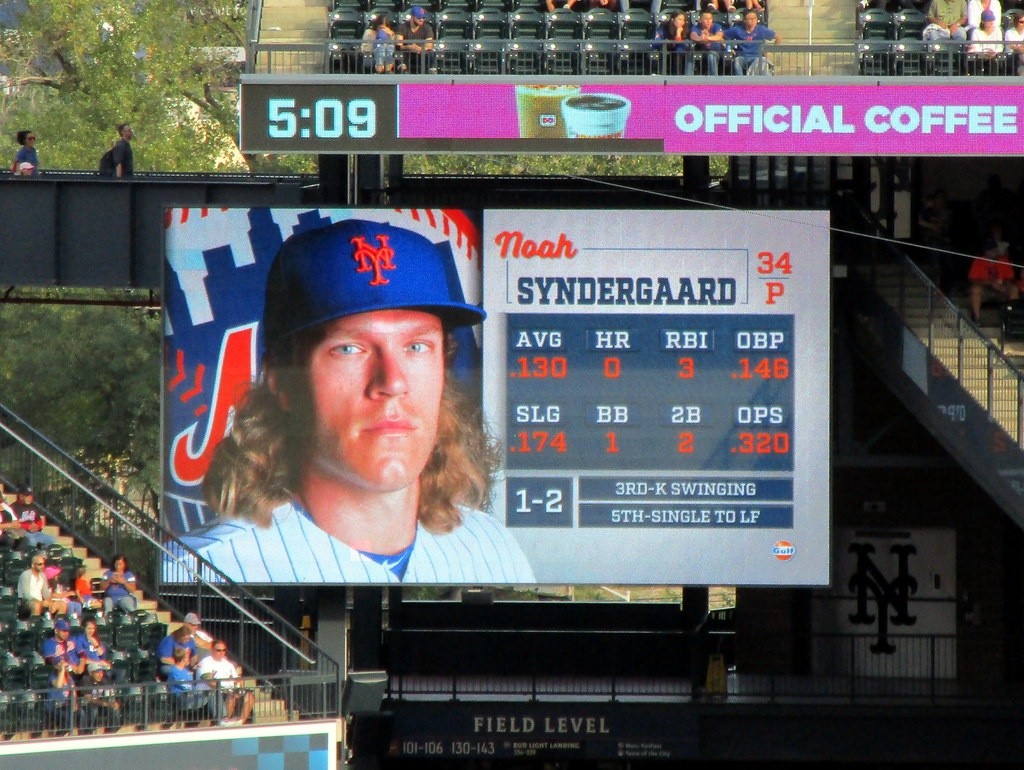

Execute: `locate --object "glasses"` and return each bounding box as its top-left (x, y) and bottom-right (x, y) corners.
top-left (215, 649), bottom-right (226, 651)
top-left (34, 563), bottom-right (44, 565)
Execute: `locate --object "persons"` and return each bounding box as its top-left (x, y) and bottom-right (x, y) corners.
top-left (1004, 12), bottom-right (1024, 76)
top-left (546, 0), bottom-right (577, 14)
top-left (589, 0), bottom-right (618, 12)
top-left (721, 8), bottom-right (783, 77)
top-left (745, 0), bottom-right (765, 12)
top-left (12, 130), bottom-right (39, 175)
top-left (689, 10), bottom-right (723, 75)
top-left (162, 218), bottom-right (488, 582)
top-left (658, 9), bottom-right (693, 76)
top-left (111, 122), bottom-right (133, 177)
top-left (360, 14), bottom-right (396, 74)
top-left (706, 0), bottom-right (736, 13)
top-left (919, 191), bottom-right (1024, 327)
top-left (968, 0), bottom-right (1001, 28)
top-left (970, 10), bottom-right (1004, 76)
top-left (395, 7), bottom-right (438, 74)
top-left (0, 480), bottom-right (255, 728)
top-left (926, 0), bottom-right (968, 42)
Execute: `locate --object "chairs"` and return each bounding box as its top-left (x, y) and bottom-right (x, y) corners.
top-left (0, 536), bottom-right (252, 731)
top-left (858, 8), bottom-right (1024, 76)
top-left (328, 0), bottom-right (768, 72)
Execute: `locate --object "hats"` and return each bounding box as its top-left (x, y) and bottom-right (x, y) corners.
top-left (981, 10), bottom-right (995, 22)
top-left (411, 7), bottom-right (426, 18)
top-left (55, 620), bottom-right (70, 630)
top-left (87, 663), bottom-right (103, 671)
top-left (53, 656), bottom-right (68, 665)
top-left (20, 487), bottom-right (34, 495)
top-left (263, 220), bottom-right (488, 343)
top-left (184, 613), bottom-right (202, 625)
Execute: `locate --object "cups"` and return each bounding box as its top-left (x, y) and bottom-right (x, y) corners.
top-left (514, 84), bottom-right (582, 138)
top-left (562, 94), bottom-right (630, 139)
